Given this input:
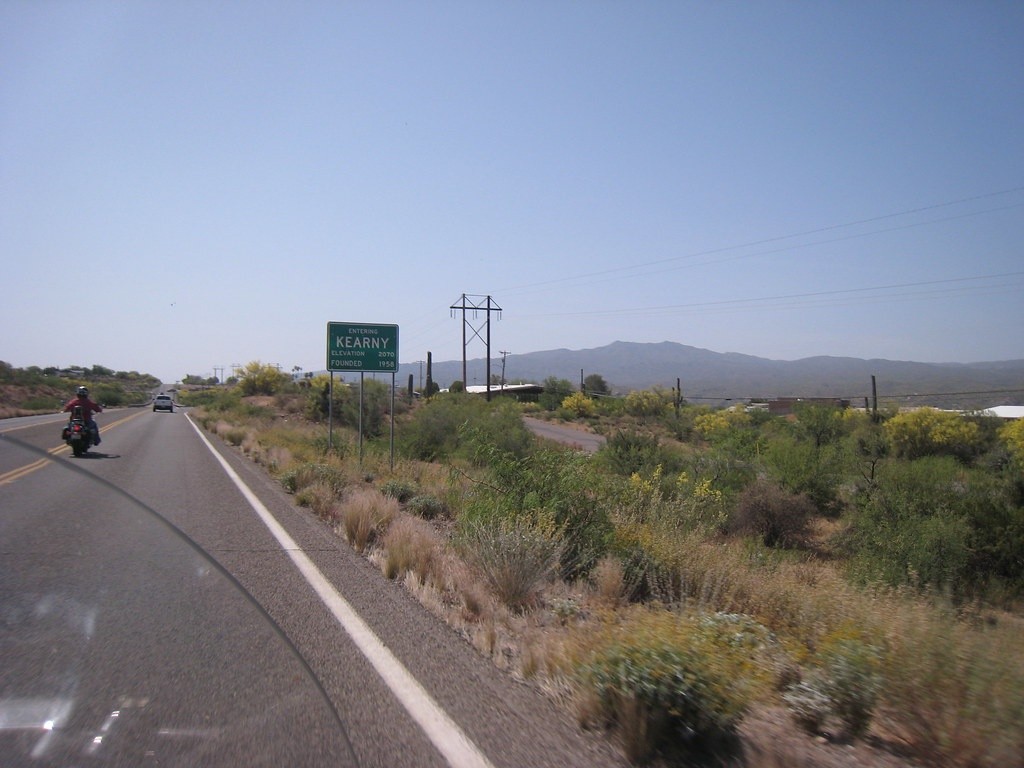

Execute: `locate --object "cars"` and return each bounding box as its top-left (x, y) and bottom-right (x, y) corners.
top-left (152, 393), bottom-right (173, 412)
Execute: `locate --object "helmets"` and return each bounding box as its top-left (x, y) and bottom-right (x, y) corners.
top-left (76, 387), bottom-right (88, 395)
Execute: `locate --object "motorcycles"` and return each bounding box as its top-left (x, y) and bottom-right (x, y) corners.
top-left (60, 399), bottom-right (107, 457)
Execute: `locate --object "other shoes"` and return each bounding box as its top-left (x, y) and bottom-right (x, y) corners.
top-left (94, 438), bottom-right (101, 445)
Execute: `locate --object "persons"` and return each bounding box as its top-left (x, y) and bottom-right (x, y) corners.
top-left (62, 386), bottom-right (102, 446)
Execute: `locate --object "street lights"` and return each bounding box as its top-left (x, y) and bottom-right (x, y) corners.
top-left (490, 351), bottom-right (511, 396)
top-left (410, 360), bottom-right (426, 396)
